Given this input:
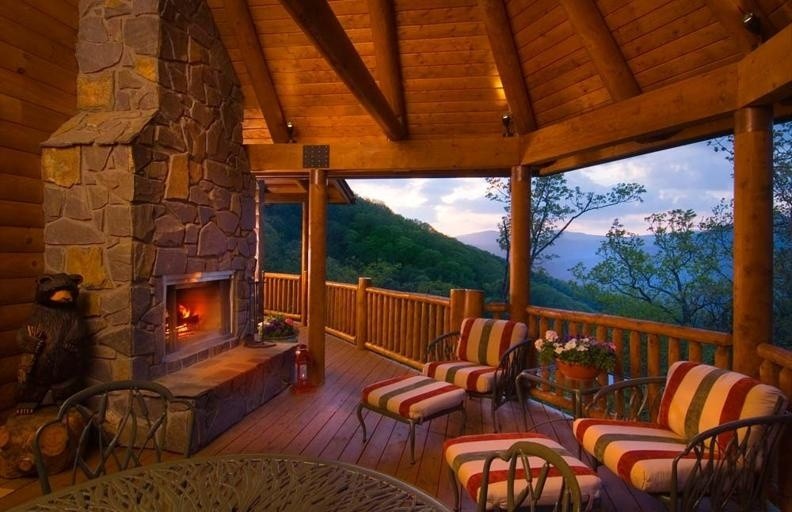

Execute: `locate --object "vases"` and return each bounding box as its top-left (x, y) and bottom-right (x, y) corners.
top-left (552, 355), bottom-right (606, 382)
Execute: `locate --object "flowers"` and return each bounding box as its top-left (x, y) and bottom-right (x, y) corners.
top-left (258, 309), bottom-right (302, 337)
top-left (534, 329), bottom-right (618, 374)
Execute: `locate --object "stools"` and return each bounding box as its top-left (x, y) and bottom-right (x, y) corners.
top-left (356, 374), bottom-right (467, 465)
top-left (443, 431), bottom-right (602, 512)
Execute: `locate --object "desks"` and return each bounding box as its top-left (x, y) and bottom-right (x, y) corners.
top-left (4, 452), bottom-right (453, 511)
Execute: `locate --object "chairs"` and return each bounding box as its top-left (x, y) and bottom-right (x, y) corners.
top-left (571, 360), bottom-right (791, 511)
top-left (32, 380), bottom-right (197, 496)
top-left (476, 441), bottom-right (582, 512)
top-left (421, 318), bottom-right (532, 434)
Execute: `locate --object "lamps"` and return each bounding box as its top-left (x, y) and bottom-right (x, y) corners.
top-left (285, 125), bottom-right (297, 143)
top-left (501, 116), bottom-right (514, 137)
top-left (742, 12), bottom-right (761, 33)
